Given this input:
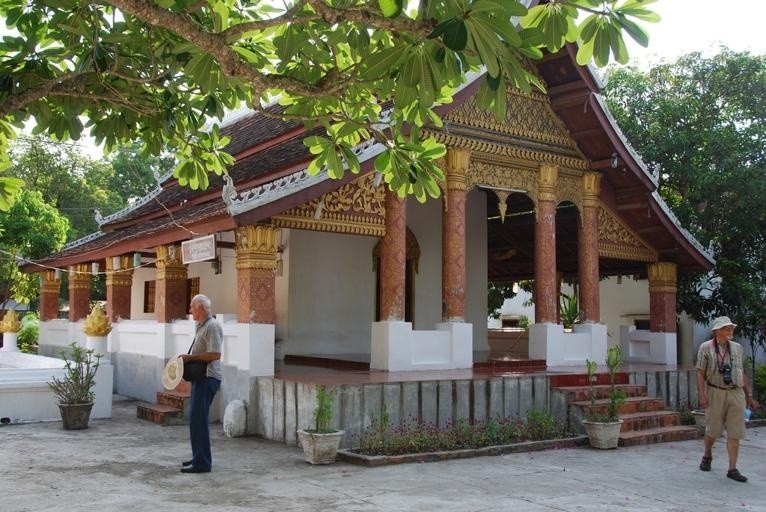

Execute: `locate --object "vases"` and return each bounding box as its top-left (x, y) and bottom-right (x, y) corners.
top-left (691, 409), bottom-right (707, 436)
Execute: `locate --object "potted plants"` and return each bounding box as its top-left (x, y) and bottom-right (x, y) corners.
top-left (582, 344), bottom-right (627, 450)
top-left (47, 341), bottom-right (104, 430)
top-left (296, 384), bottom-right (346, 465)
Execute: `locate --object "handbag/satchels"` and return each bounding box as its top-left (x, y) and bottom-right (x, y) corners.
top-left (184, 361), bottom-right (207, 382)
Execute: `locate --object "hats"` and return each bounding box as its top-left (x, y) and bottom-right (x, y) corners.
top-left (161, 356), bottom-right (185, 391)
top-left (711, 316), bottom-right (738, 331)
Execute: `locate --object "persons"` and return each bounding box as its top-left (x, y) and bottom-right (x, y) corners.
top-left (695, 316), bottom-right (758, 482)
top-left (176, 294), bottom-right (225, 474)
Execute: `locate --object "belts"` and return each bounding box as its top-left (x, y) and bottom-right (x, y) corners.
top-left (707, 382), bottom-right (737, 390)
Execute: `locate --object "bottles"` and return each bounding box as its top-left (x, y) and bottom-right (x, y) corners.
top-left (744, 405), bottom-right (752, 422)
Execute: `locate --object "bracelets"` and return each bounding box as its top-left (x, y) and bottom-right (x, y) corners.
top-left (746, 393), bottom-right (753, 397)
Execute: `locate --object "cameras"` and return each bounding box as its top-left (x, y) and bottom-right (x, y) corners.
top-left (719, 364), bottom-right (732, 385)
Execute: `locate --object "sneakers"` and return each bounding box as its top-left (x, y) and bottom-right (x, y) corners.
top-left (727, 469), bottom-right (747, 481)
top-left (180, 459), bottom-right (211, 473)
top-left (700, 456), bottom-right (712, 471)
